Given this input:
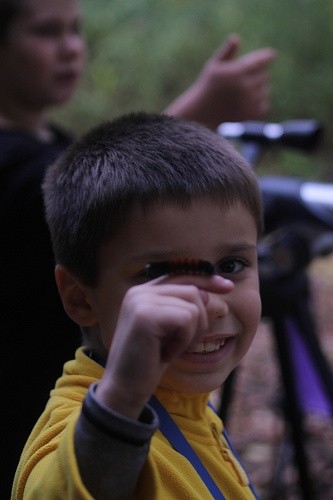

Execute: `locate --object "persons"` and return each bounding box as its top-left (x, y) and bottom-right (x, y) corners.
top-left (0, 0), bottom-right (278, 500)
top-left (207, 252), bottom-right (333, 500)
top-left (8, 110), bottom-right (260, 500)
